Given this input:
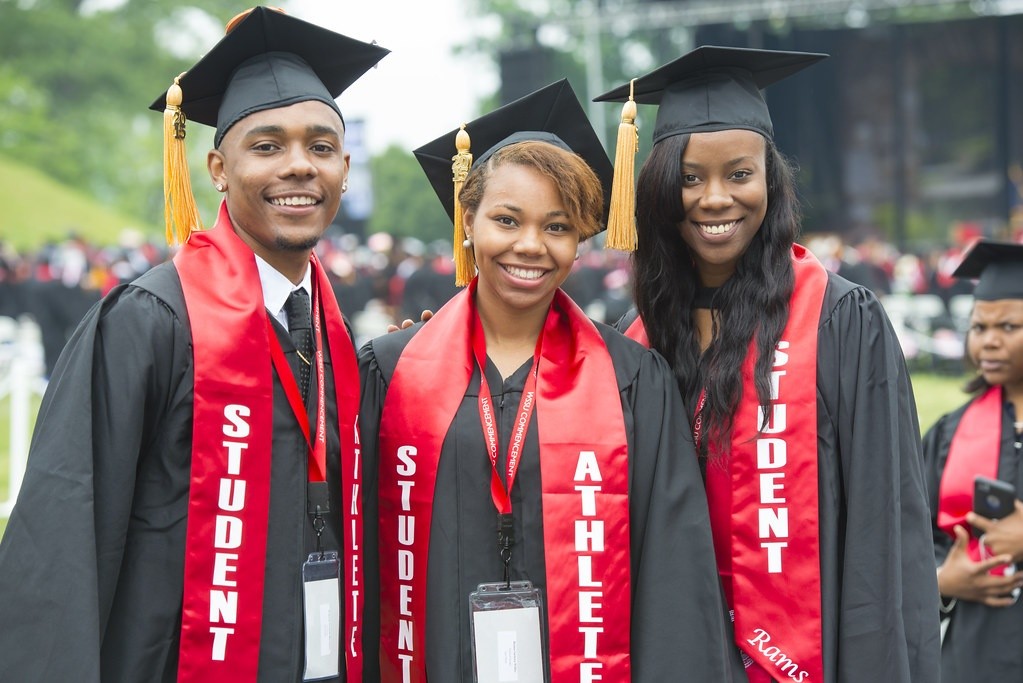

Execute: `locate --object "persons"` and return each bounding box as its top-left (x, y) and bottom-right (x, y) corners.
top-left (387, 45), bottom-right (943, 683)
top-left (0, 231), bottom-right (1023, 386)
top-left (0, 8), bottom-right (391, 683)
top-left (922, 238), bottom-right (1023, 683)
top-left (354, 78), bottom-right (749, 683)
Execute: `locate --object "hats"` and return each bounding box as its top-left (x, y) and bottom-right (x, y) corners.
top-left (950, 241), bottom-right (1023, 300)
top-left (592, 46), bottom-right (830, 252)
top-left (150, 5), bottom-right (392, 249)
top-left (412, 78), bottom-right (615, 288)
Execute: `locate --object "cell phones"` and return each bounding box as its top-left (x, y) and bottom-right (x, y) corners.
top-left (972, 478), bottom-right (1016, 539)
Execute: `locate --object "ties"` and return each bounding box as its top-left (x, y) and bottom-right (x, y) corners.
top-left (283, 287), bottom-right (312, 407)
